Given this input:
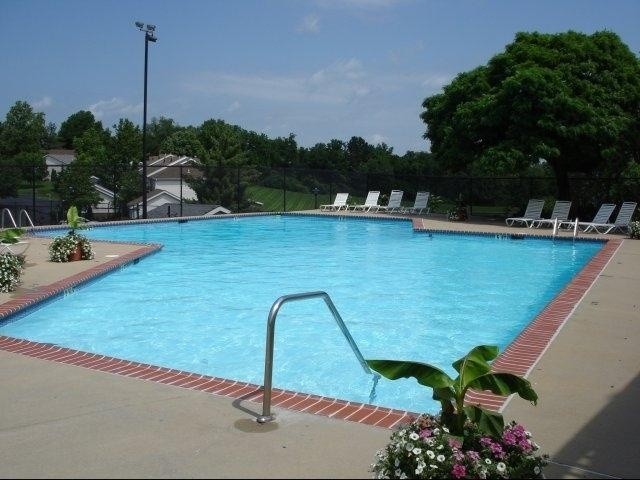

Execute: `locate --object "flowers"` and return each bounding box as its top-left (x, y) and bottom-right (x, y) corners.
top-left (628, 221), bottom-right (640, 240)
top-left (0, 252), bottom-right (26, 292)
top-left (367, 413), bottom-right (549, 479)
top-left (47, 234), bottom-right (95, 262)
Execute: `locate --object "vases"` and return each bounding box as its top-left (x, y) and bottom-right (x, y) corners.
top-left (69, 244), bottom-right (82, 260)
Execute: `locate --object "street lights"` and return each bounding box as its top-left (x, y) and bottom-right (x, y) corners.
top-left (135, 21), bottom-right (157, 218)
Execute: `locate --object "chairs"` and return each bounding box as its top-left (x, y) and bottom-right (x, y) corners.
top-left (319, 190), bottom-right (431, 216)
top-left (0, 237), bottom-right (30, 255)
top-left (505, 198), bottom-right (637, 234)
